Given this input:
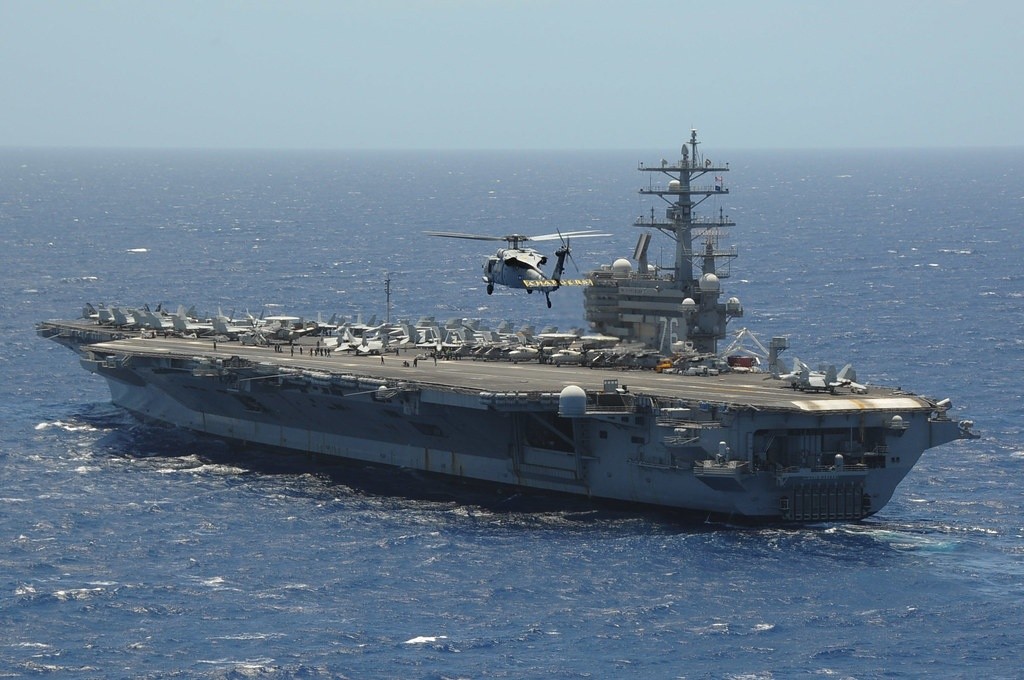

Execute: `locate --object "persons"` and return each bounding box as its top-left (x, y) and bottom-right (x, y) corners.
top-left (402, 357), bottom-right (417, 367)
top-left (381, 355), bottom-right (384, 363)
top-left (164, 331), bottom-right (167, 339)
top-left (213, 341), bottom-right (217, 349)
top-left (274, 343), bottom-right (280, 351)
top-left (290, 343), bottom-right (330, 356)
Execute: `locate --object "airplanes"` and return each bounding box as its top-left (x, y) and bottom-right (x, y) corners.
top-left (762, 357), bottom-right (868, 397)
top-left (84, 302), bottom-right (621, 368)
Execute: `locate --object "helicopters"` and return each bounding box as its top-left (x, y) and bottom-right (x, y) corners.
top-left (421, 228), bottom-right (615, 308)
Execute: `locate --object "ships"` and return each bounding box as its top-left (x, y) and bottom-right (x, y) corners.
top-left (34, 128), bottom-right (983, 523)
